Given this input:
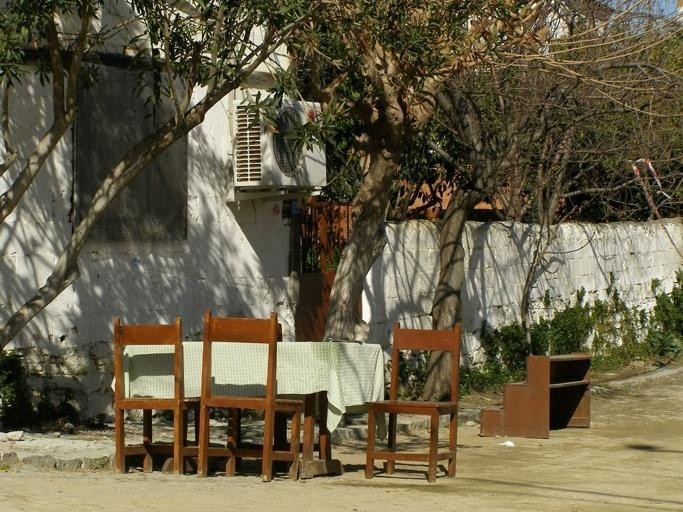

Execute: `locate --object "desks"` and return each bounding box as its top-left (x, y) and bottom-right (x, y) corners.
top-left (111, 341), bottom-right (386, 477)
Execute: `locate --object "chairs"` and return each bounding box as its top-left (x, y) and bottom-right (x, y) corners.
top-left (196, 310), bottom-right (304, 482)
top-left (364, 321), bottom-right (461, 483)
top-left (113, 318), bottom-right (201, 475)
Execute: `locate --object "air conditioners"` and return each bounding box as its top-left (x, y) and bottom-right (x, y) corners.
top-left (223, 100), bottom-right (327, 203)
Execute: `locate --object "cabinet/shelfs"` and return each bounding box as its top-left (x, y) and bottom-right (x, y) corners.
top-left (480, 352), bottom-right (593, 439)
